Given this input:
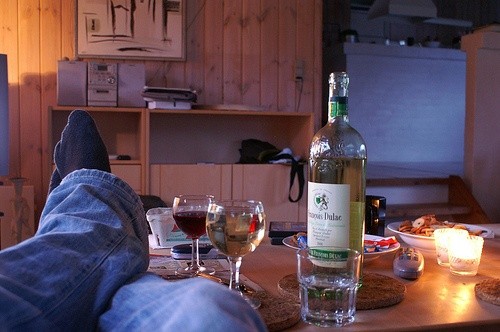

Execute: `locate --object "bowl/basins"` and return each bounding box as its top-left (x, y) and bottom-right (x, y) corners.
top-left (146, 205), bottom-right (214, 248)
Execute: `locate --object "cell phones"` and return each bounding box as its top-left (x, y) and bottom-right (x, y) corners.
top-left (170, 243), bottom-right (218, 259)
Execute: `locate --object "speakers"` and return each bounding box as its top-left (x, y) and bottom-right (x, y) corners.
top-left (56, 56), bottom-right (147, 109)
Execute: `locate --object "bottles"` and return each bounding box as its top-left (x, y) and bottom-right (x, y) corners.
top-left (307, 73), bottom-right (367, 300)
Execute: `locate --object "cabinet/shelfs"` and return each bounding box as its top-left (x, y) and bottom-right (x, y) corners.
top-left (45, 106), bottom-right (145, 199)
top-left (145, 112), bottom-right (316, 224)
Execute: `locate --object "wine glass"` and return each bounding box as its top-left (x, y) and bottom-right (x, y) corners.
top-left (205, 199), bottom-right (266, 312)
top-left (171, 194), bottom-right (217, 279)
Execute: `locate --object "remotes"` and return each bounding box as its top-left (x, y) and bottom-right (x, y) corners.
top-left (392, 246), bottom-right (425, 278)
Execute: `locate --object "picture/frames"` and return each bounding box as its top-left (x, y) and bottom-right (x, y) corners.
top-left (72, 1), bottom-right (188, 64)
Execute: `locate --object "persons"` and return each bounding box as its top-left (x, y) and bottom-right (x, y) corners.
top-left (0, 109), bottom-right (267, 332)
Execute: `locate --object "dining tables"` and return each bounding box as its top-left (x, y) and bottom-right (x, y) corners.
top-left (142, 222), bottom-right (500, 329)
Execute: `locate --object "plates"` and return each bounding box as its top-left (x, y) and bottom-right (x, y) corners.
top-left (281, 234), bottom-right (400, 261)
top-left (386, 221), bottom-right (491, 248)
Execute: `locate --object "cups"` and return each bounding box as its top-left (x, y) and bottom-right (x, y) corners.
top-left (433, 228), bottom-right (470, 267)
top-left (296, 245), bottom-right (362, 328)
top-left (447, 233), bottom-right (484, 275)
